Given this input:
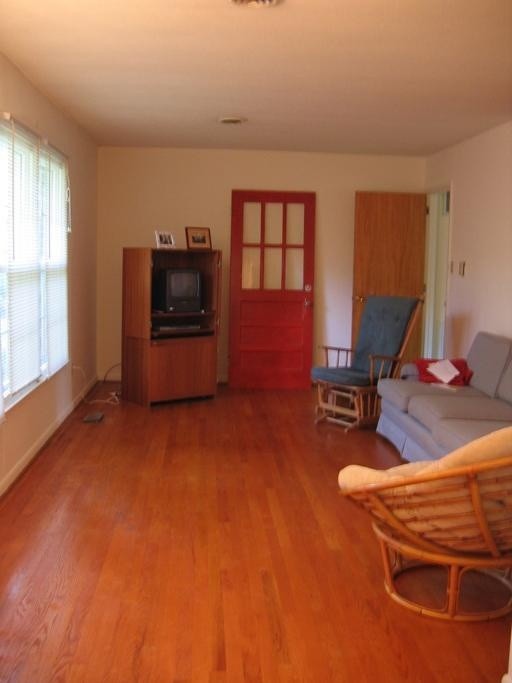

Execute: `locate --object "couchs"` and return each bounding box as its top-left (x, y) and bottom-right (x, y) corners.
top-left (376, 330), bottom-right (511, 463)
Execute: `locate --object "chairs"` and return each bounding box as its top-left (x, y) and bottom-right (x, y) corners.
top-left (309, 294), bottom-right (427, 433)
top-left (337, 425), bottom-right (512, 622)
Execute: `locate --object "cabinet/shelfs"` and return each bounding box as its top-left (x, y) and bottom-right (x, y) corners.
top-left (121, 246), bottom-right (222, 407)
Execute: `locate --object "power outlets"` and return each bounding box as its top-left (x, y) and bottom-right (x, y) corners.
top-left (69, 363), bottom-right (74, 377)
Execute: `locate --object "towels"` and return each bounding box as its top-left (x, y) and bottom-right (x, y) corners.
top-left (426, 358), bottom-right (460, 384)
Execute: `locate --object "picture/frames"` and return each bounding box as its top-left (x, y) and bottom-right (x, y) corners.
top-left (154, 229), bottom-right (175, 249)
top-left (185, 226), bottom-right (212, 250)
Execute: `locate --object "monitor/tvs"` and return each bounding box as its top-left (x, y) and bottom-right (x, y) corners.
top-left (154, 266), bottom-right (202, 314)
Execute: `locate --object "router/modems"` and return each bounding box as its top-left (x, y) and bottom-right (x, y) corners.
top-left (83, 411), bottom-right (104, 424)
